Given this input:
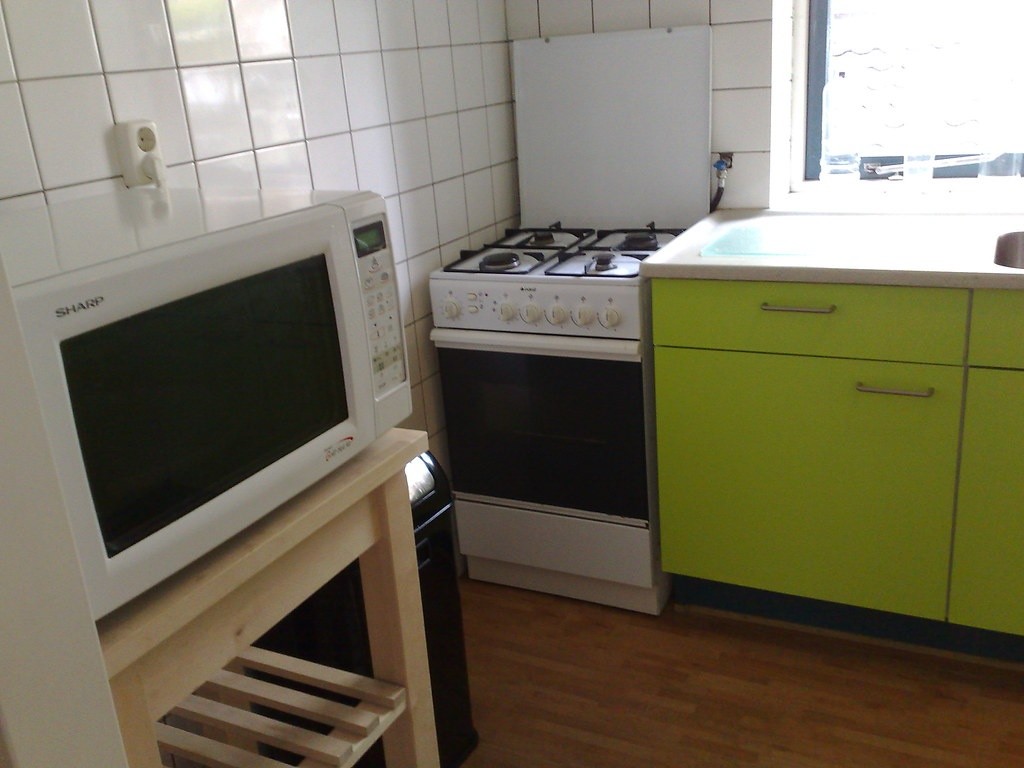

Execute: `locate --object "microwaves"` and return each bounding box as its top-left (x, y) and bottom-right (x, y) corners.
top-left (10, 192), bottom-right (412, 623)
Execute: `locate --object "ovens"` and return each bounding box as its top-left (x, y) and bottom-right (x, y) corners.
top-left (429, 327), bottom-right (676, 614)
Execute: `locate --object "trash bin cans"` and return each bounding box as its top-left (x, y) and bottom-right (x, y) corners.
top-left (245, 451), bottom-right (481, 768)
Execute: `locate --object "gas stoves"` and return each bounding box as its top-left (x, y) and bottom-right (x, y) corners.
top-left (429, 220), bottom-right (686, 339)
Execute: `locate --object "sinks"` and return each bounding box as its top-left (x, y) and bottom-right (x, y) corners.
top-left (991, 229), bottom-right (1023, 269)
